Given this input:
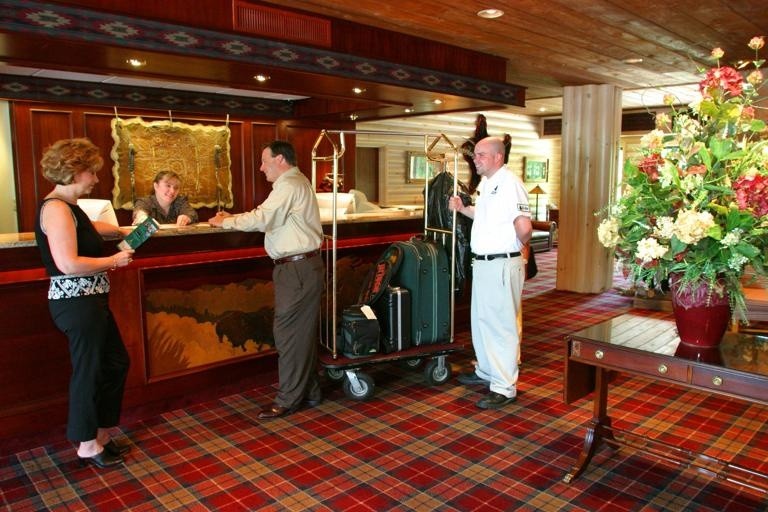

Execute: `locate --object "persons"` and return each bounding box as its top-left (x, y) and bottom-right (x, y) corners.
top-left (207, 141), bottom-right (325, 420)
top-left (131, 169), bottom-right (199, 228)
top-left (449, 137), bottom-right (533, 410)
top-left (34, 138), bottom-right (135, 464)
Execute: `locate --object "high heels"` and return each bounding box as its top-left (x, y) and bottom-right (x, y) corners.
top-left (78, 439), bottom-right (129, 467)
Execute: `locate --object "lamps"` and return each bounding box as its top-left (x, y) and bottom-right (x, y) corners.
top-left (528, 184), bottom-right (547, 218)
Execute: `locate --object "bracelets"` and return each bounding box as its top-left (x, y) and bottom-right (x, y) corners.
top-left (110, 258), bottom-right (117, 271)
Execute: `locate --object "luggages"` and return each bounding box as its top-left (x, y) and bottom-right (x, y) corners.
top-left (392, 233), bottom-right (451, 348)
top-left (378, 282), bottom-right (412, 355)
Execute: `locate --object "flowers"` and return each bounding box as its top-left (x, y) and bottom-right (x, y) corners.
top-left (595, 34), bottom-right (768, 328)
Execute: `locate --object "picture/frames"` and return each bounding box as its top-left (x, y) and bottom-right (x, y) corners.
top-left (406, 151), bottom-right (448, 184)
top-left (523, 156), bottom-right (549, 183)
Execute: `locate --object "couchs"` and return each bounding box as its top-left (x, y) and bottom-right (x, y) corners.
top-left (527, 219), bottom-right (558, 253)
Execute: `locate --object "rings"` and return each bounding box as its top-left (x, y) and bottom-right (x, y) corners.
top-left (128, 257), bottom-right (134, 262)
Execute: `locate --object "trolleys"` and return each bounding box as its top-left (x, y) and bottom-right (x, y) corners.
top-left (310, 129), bottom-right (465, 403)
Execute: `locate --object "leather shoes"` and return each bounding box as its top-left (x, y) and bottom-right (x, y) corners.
top-left (304, 399), bottom-right (320, 407)
top-left (257, 403), bottom-right (296, 419)
top-left (476, 391), bottom-right (517, 409)
top-left (457, 371), bottom-right (488, 383)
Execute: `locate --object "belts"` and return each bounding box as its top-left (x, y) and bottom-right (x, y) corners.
top-left (469, 252), bottom-right (521, 260)
top-left (272, 252), bottom-right (317, 265)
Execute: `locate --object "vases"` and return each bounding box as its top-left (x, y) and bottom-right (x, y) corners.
top-left (669, 273), bottom-right (732, 348)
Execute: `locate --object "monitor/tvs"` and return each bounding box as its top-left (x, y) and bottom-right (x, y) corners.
top-left (316, 193), bottom-right (355, 215)
top-left (76, 199), bottom-right (119, 227)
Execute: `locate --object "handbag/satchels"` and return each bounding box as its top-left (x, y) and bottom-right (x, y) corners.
top-left (336, 307), bottom-right (378, 359)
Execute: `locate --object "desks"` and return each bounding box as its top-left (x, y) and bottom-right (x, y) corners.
top-left (563, 308), bottom-right (768, 506)
